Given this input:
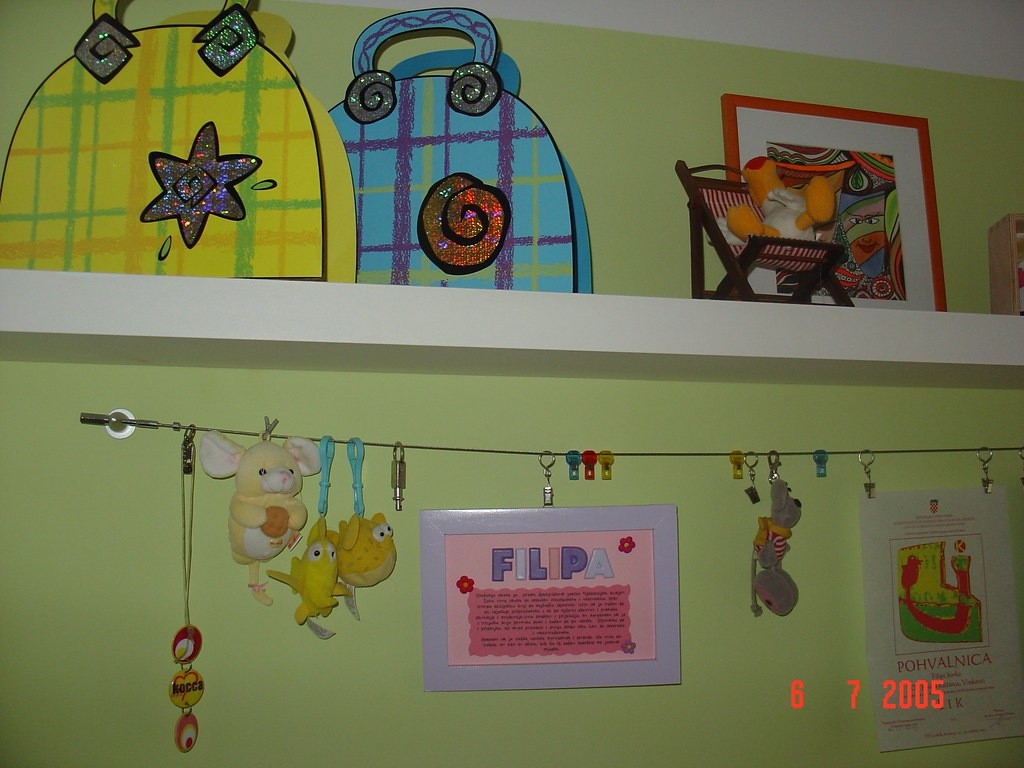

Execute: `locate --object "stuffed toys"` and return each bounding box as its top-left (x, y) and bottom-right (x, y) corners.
top-left (705, 156), bottom-right (834, 245)
top-left (266, 517), bottom-right (348, 626)
top-left (324, 513), bottom-right (397, 588)
top-left (199, 429), bottom-right (322, 606)
top-left (750, 479), bottom-right (802, 616)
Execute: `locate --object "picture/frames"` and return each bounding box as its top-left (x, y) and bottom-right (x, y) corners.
top-left (420, 502), bottom-right (680, 692)
top-left (720, 93), bottom-right (948, 313)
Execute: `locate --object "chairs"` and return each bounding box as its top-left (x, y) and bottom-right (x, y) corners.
top-left (673, 159), bottom-right (856, 308)
